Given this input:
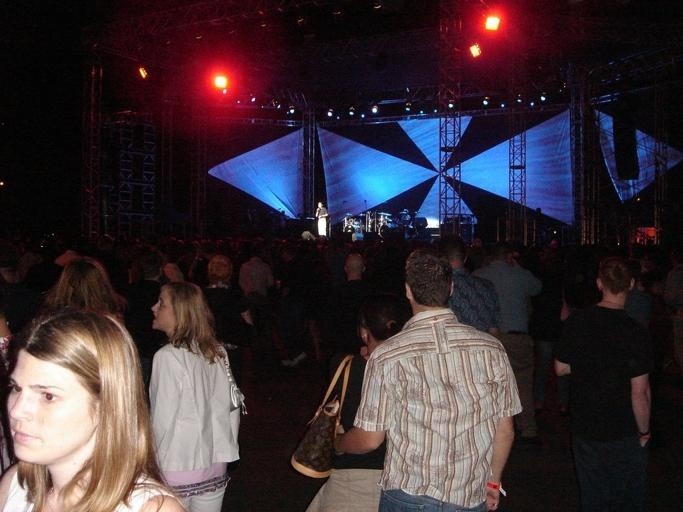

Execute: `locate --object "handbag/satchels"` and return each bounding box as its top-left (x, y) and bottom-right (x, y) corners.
top-left (212, 344), bottom-right (250, 417)
top-left (291, 353), bottom-right (352, 479)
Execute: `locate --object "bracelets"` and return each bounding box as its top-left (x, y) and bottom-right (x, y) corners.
top-left (486, 480), bottom-right (506, 498)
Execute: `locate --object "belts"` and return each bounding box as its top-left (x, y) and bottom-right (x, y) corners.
top-left (505, 330), bottom-right (529, 338)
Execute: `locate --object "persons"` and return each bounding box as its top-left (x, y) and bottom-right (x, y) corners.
top-left (315, 201), bottom-right (328, 236)
top-left (278, 244), bottom-right (302, 297)
top-left (302, 227), bottom-right (316, 240)
top-left (551, 232), bottom-right (660, 258)
top-left (0, 308), bottom-right (187, 512)
top-left (2, 248), bottom-right (77, 295)
top-left (447, 236), bottom-right (512, 338)
top-left (148, 280), bottom-right (244, 512)
top-left (305, 286), bottom-right (412, 512)
top-left (332, 247), bottom-right (525, 512)
top-left (554, 258), bottom-right (654, 512)
top-left (199, 256), bottom-right (238, 333)
top-left (332, 254), bottom-right (371, 352)
top-left (276, 239), bottom-right (443, 271)
top-left (35, 257), bottom-right (127, 328)
top-left (469, 238), bottom-right (543, 450)
top-left (238, 242), bottom-right (276, 300)
top-left (662, 248), bottom-right (682, 369)
top-left (0, 233), bottom-right (235, 267)
